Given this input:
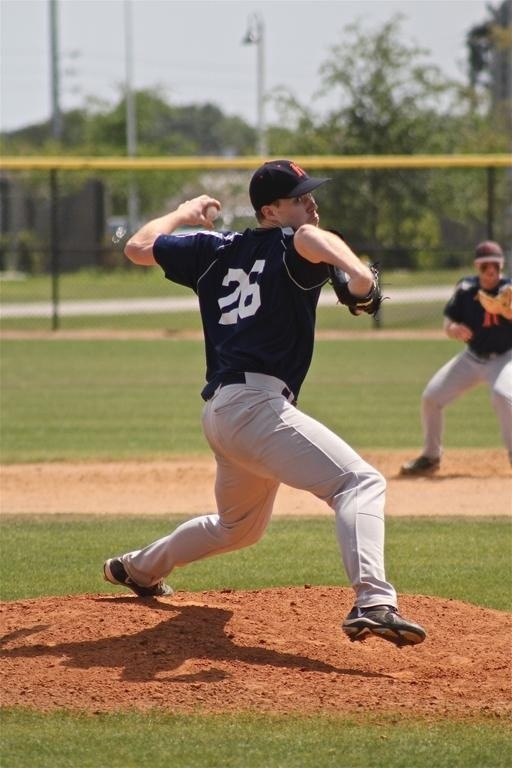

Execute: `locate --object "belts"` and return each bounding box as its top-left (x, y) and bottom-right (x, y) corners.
top-left (221, 370), bottom-right (299, 404)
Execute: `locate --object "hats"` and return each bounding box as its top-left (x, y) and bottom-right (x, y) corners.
top-left (472, 239), bottom-right (506, 264)
top-left (249, 160), bottom-right (335, 211)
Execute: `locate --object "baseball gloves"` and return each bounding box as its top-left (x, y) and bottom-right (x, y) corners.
top-left (327, 257), bottom-right (389, 320)
top-left (477, 285), bottom-right (512, 329)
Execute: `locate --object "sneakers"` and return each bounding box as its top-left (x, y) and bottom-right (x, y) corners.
top-left (102, 555), bottom-right (177, 598)
top-left (399, 450), bottom-right (442, 475)
top-left (340, 600), bottom-right (427, 645)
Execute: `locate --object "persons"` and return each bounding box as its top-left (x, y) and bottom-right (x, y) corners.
top-left (102, 161), bottom-right (427, 650)
top-left (401, 239), bottom-right (512, 476)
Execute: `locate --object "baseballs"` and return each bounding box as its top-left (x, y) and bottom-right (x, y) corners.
top-left (203, 206), bottom-right (218, 221)
